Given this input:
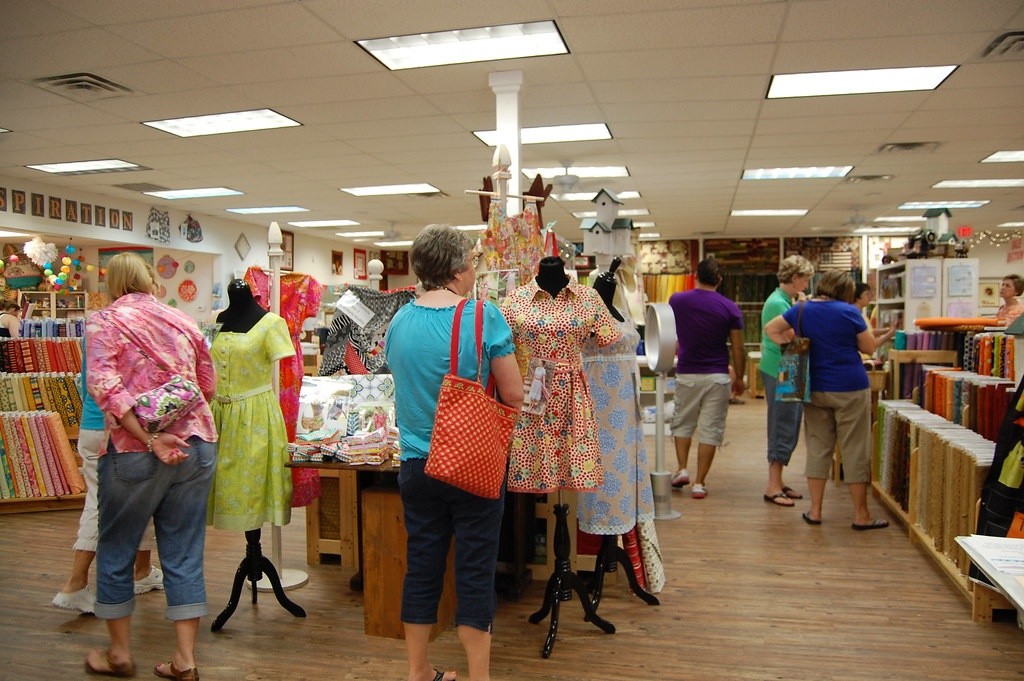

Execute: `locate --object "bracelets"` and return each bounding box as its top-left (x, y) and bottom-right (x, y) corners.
top-left (148, 433), bottom-right (158, 452)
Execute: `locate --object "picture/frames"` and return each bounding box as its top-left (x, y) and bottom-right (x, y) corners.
top-left (279, 229), bottom-right (294, 271)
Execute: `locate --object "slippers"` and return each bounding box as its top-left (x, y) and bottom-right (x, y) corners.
top-left (853, 518), bottom-right (888, 529)
top-left (803, 511), bottom-right (821, 524)
top-left (782, 487), bottom-right (803, 498)
top-left (433, 669), bottom-right (455, 681)
top-left (764, 493), bottom-right (795, 506)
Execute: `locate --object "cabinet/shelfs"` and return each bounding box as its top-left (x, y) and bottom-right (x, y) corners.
top-left (866, 259), bottom-right (980, 360)
top-left (17, 288), bottom-right (89, 322)
top-left (306, 469), bottom-right (360, 568)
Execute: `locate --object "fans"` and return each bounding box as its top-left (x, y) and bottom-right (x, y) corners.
top-left (537, 155), bottom-right (617, 193)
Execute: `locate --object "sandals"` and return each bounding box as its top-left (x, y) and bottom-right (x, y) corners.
top-left (84, 649), bottom-right (134, 676)
top-left (154, 662), bottom-right (199, 681)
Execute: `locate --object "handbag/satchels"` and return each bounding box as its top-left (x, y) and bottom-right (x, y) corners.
top-left (133, 373), bottom-right (201, 433)
top-left (424, 297), bottom-right (519, 499)
top-left (774, 301), bottom-right (811, 404)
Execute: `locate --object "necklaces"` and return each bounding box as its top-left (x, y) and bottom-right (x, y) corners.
top-left (441, 286), bottom-right (456, 294)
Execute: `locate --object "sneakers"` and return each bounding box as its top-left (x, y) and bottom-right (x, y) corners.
top-left (52, 585), bottom-right (96, 613)
top-left (134, 566), bottom-right (164, 594)
top-left (671, 470), bottom-right (690, 488)
top-left (692, 482), bottom-right (707, 497)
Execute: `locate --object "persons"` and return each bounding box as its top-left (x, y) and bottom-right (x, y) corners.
top-left (765, 267), bottom-right (890, 530)
top-left (49, 338), bottom-right (164, 612)
top-left (384, 223), bottom-right (523, 680)
top-left (0, 304), bottom-right (20, 337)
top-left (85, 252), bottom-right (217, 681)
top-left (668, 259), bottom-right (744, 499)
top-left (207, 278), bottom-right (306, 531)
top-left (527, 360), bottom-right (546, 411)
top-left (997, 275), bottom-right (1024, 325)
top-left (759, 255), bottom-right (815, 507)
top-left (502, 257), bottom-right (620, 493)
top-left (855, 284), bottom-right (870, 312)
top-left (580, 257), bottom-right (660, 535)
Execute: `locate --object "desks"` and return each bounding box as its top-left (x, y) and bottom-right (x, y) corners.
top-left (284, 461), bottom-right (457, 642)
